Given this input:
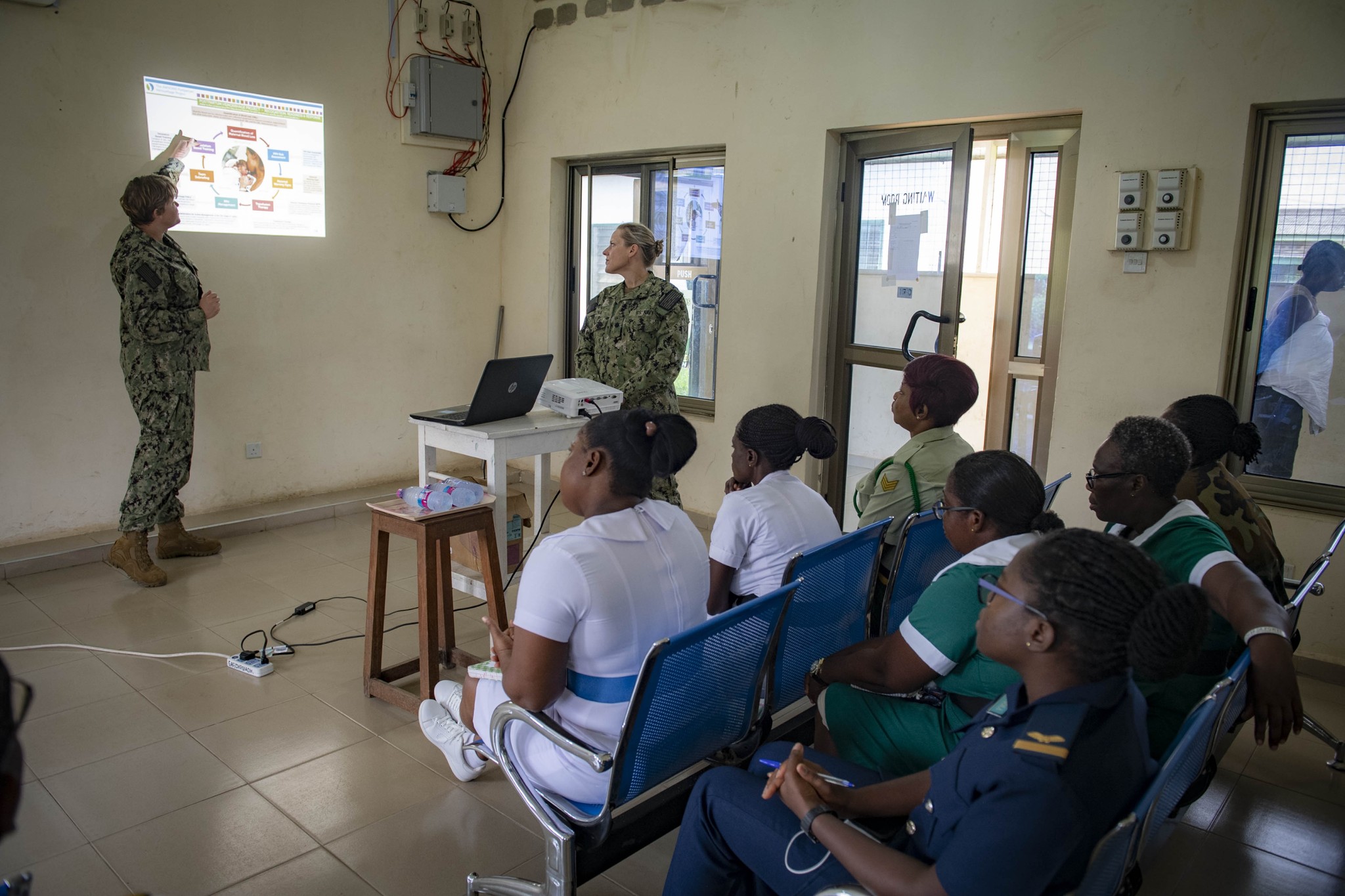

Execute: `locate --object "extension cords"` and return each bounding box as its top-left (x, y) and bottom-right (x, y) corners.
top-left (227, 653), bottom-right (274, 677)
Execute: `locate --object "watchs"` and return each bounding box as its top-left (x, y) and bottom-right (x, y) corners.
top-left (809, 658), bottom-right (827, 686)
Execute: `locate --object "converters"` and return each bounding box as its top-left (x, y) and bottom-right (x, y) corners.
top-left (294, 601), bottom-right (316, 615)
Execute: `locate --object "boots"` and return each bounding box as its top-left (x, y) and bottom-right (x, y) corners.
top-left (103, 529), bottom-right (168, 587)
top-left (156, 517), bottom-right (222, 558)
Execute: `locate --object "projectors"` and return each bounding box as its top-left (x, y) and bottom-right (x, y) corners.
top-left (537, 378), bottom-right (623, 419)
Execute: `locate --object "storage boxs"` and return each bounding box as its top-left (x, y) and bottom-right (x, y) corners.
top-left (448, 475), bottom-right (531, 577)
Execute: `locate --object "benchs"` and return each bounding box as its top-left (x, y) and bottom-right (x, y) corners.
top-left (467, 472), bottom-right (1344, 896)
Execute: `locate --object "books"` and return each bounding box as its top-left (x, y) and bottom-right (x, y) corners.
top-left (467, 659), bottom-right (503, 681)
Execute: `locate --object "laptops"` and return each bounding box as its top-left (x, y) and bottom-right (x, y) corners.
top-left (410, 354), bottom-right (554, 426)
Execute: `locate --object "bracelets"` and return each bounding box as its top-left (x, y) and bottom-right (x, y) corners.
top-left (800, 804), bottom-right (839, 844)
top-left (1244, 626), bottom-right (1301, 653)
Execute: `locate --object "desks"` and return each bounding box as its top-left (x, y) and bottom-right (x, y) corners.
top-left (419, 407), bottom-right (598, 602)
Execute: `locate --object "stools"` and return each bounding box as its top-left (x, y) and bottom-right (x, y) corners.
top-left (365, 499), bottom-right (507, 712)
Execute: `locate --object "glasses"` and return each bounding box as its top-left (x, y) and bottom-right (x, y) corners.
top-left (0, 678), bottom-right (33, 755)
top-left (1086, 469), bottom-right (1138, 488)
top-left (932, 501), bottom-right (978, 519)
top-left (976, 573), bottom-right (1047, 620)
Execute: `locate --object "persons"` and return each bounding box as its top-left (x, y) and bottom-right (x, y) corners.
top-left (418, 409), bottom-right (711, 805)
top-left (805, 449), bottom-right (1065, 773)
top-left (107, 138), bottom-right (223, 588)
top-left (573, 222), bottom-right (690, 511)
top-left (1085, 415), bottom-right (1303, 751)
top-left (853, 353), bottom-right (976, 530)
top-left (1159, 394), bottom-right (1290, 607)
top-left (706, 404), bottom-right (843, 616)
top-left (1246, 240), bottom-right (1345, 479)
top-left (662, 527), bottom-right (1208, 896)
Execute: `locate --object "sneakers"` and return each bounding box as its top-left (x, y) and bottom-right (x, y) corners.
top-left (419, 699), bottom-right (486, 781)
top-left (434, 680), bottom-right (473, 732)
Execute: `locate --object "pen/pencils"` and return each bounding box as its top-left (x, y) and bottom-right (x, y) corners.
top-left (760, 760), bottom-right (859, 790)
